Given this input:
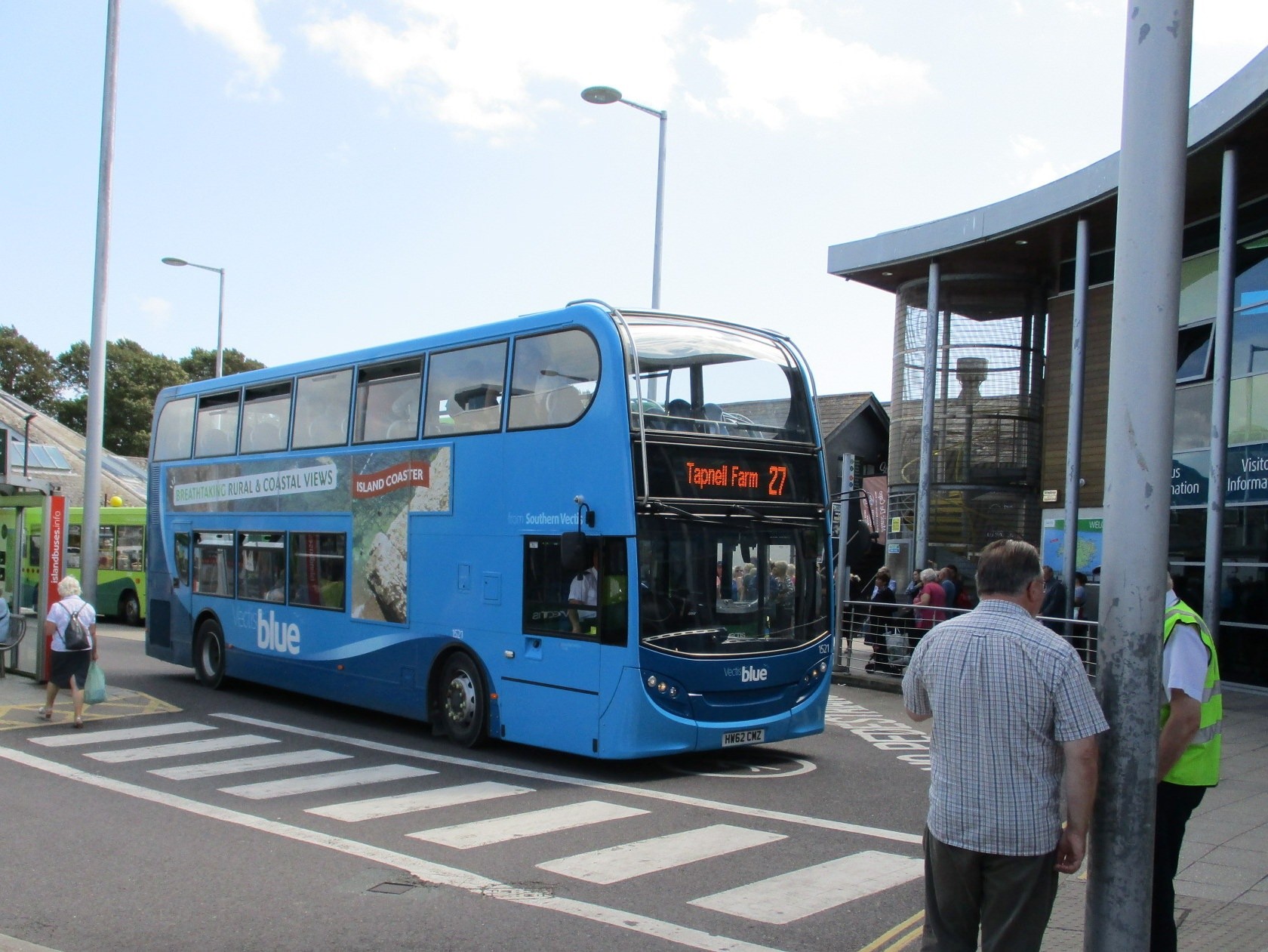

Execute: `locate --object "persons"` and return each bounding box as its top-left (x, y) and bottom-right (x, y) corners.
top-left (901, 538), bottom-right (1110, 952)
top-left (1150, 559), bottom-right (1223, 952)
top-left (264, 561), bottom-right (344, 608)
top-left (1035, 565), bottom-right (1088, 663)
top-left (568, 550), bottom-right (622, 638)
top-left (34, 576), bottom-right (99, 729)
top-left (717, 557), bottom-right (963, 673)
top-left (0, 589), bottom-right (10, 643)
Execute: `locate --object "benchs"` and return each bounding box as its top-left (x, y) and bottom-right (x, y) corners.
top-left (0, 614), bottom-right (26, 678)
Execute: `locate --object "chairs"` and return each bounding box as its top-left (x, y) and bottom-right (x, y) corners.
top-left (630, 397), bottom-right (766, 438)
top-left (181, 386), bottom-right (585, 457)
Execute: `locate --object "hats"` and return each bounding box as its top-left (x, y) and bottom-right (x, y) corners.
top-left (717, 560), bottom-right (722, 565)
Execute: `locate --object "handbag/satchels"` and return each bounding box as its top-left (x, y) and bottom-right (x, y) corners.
top-left (903, 633), bottom-right (912, 654)
top-left (885, 628), bottom-right (910, 666)
top-left (1073, 607), bottom-right (1080, 620)
top-left (83, 659), bottom-right (107, 704)
top-left (862, 617), bottom-right (870, 636)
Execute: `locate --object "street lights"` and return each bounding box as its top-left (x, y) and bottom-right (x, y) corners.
top-left (161, 257), bottom-right (226, 429)
top-left (23, 413), bottom-right (38, 492)
top-left (580, 84), bottom-right (668, 404)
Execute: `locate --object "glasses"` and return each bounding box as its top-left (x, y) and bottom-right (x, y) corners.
top-left (1027, 580), bottom-right (1047, 593)
top-left (736, 570), bottom-right (743, 572)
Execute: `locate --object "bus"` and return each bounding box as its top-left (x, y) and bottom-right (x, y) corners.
top-left (144, 297), bottom-right (880, 763)
top-left (0, 507), bottom-right (146, 627)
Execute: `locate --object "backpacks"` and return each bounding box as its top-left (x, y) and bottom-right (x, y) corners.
top-left (54, 602), bottom-right (91, 650)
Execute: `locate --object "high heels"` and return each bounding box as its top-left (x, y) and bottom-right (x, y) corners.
top-left (38, 707), bottom-right (52, 719)
top-left (73, 716), bottom-right (84, 729)
